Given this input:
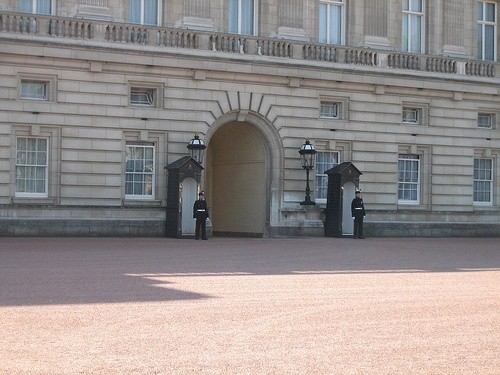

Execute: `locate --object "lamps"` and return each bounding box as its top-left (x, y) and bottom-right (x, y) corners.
top-left (298, 140), bottom-right (316, 170)
top-left (187, 131), bottom-right (206, 164)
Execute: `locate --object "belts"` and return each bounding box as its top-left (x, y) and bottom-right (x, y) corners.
top-left (355, 208), bottom-right (363, 210)
top-left (197, 209), bottom-right (206, 211)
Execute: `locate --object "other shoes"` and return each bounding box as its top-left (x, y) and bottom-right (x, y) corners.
top-left (359, 236), bottom-right (365, 238)
top-left (195, 237), bottom-right (198, 240)
top-left (353, 235), bottom-right (357, 239)
top-left (202, 238), bottom-right (208, 240)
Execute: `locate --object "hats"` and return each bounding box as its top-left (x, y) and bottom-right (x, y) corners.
top-left (199, 191), bottom-right (205, 195)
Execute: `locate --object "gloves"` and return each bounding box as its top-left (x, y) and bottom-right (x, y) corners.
top-left (194, 218), bottom-right (196, 221)
top-left (363, 216), bottom-right (365, 218)
top-left (207, 218), bottom-right (209, 220)
top-left (352, 217), bottom-right (355, 220)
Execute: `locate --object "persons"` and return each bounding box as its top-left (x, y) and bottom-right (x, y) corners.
top-left (193, 193), bottom-right (209, 240)
top-left (351, 190), bottom-right (366, 239)
